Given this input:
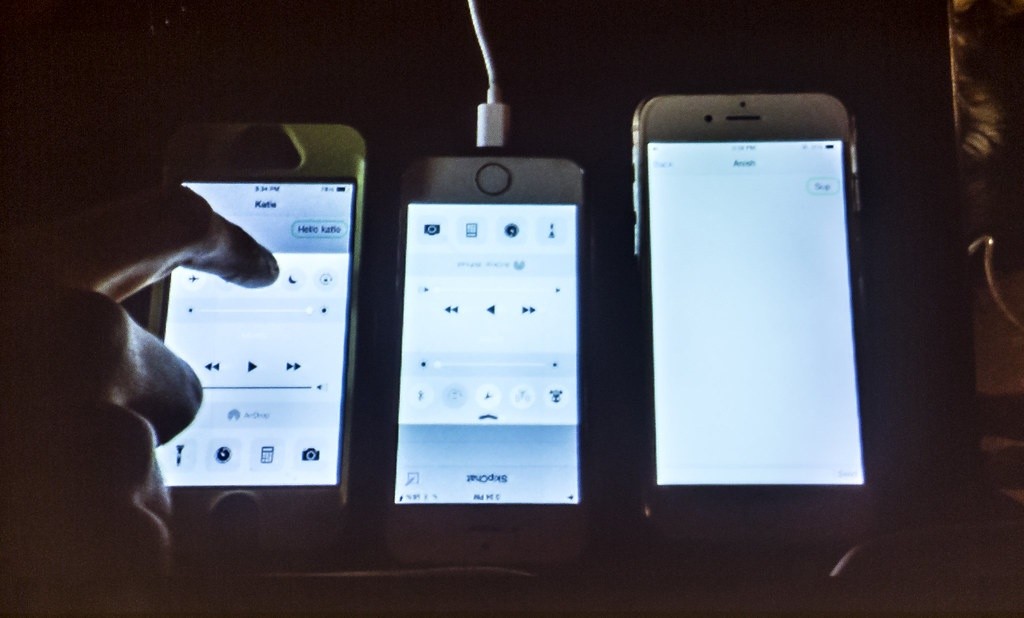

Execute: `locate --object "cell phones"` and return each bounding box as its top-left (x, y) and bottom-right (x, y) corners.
top-left (143, 123), bottom-right (365, 555)
top-left (632, 92), bottom-right (876, 545)
top-left (387, 157), bottom-right (589, 572)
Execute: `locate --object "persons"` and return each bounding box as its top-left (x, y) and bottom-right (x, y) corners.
top-left (0, 185), bottom-right (277, 574)
